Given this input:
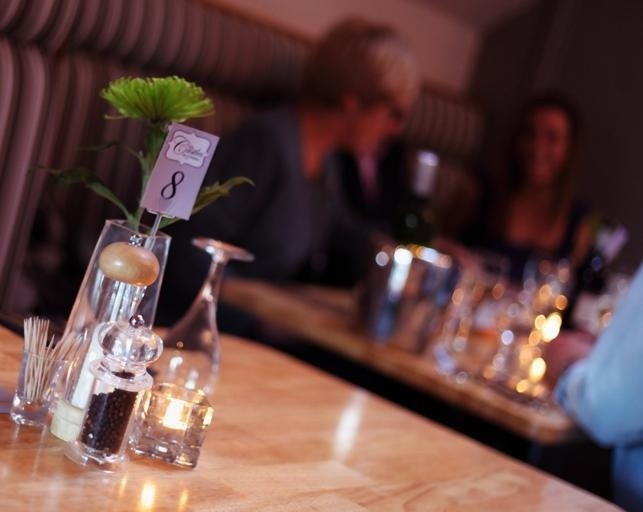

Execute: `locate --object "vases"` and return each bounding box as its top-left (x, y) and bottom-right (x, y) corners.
top-left (43, 220), bottom-right (171, 414)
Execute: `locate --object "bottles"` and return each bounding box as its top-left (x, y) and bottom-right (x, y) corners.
top-left (386, 149), bottom-right (439, 242)
top-left (66, 314), bottom-right (163, 475)
top-left (547, 198), bottom-right (629, 331)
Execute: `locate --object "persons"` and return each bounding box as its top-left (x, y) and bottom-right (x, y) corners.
top-left (538, 262), bottom-right (643, 512)
top-left (430, 95), bottom-right (608, 332)
top-left (151, 15), bottom-right (430, 323)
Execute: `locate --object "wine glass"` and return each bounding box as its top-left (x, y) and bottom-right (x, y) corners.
top-left (153, 236), bottom-right (259, 396)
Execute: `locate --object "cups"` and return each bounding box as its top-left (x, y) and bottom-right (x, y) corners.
top-left (12, 348), bottom-right (67, 428)
top-left (132, 383), bottom-right (213, 466)
